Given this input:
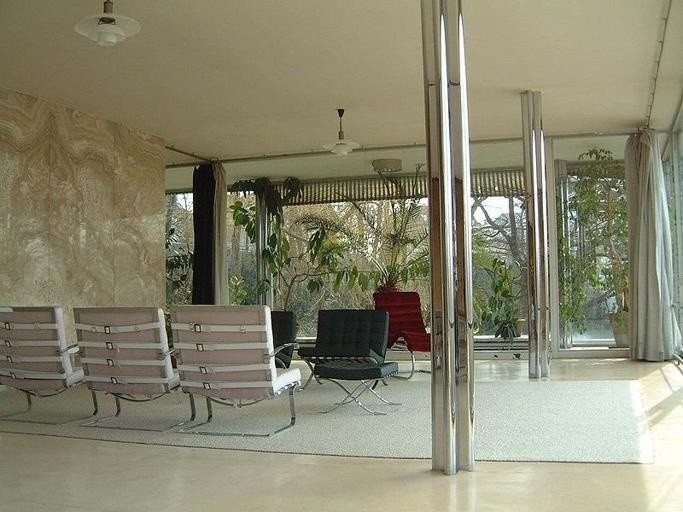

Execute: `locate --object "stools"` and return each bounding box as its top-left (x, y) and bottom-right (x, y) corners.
top-left (314, 360), bottom-right (402, 416)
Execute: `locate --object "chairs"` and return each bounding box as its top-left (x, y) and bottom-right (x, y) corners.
top-left (372, 291), bottom-right (433, 380)
top-left (0, 305), bottom-right (97, 425)
top-left (70, 305), bottom-right (196, 433)
top-left (271, 311), bottom-right (296, 367)
top-left (175, 305), bottom-right (302, 439)
top-left (297, 308), bottom-right (391, 390)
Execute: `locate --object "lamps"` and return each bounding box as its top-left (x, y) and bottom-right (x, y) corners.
top-left (72, 0), bottom-right (142, 47)
top-left (372, 158), bottom-right (402, 172)
top-left (321, 110), bottom-right (362, 156)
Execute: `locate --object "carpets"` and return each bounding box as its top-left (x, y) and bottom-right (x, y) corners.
top-left (0, 378), bottom-right (650, 464)
